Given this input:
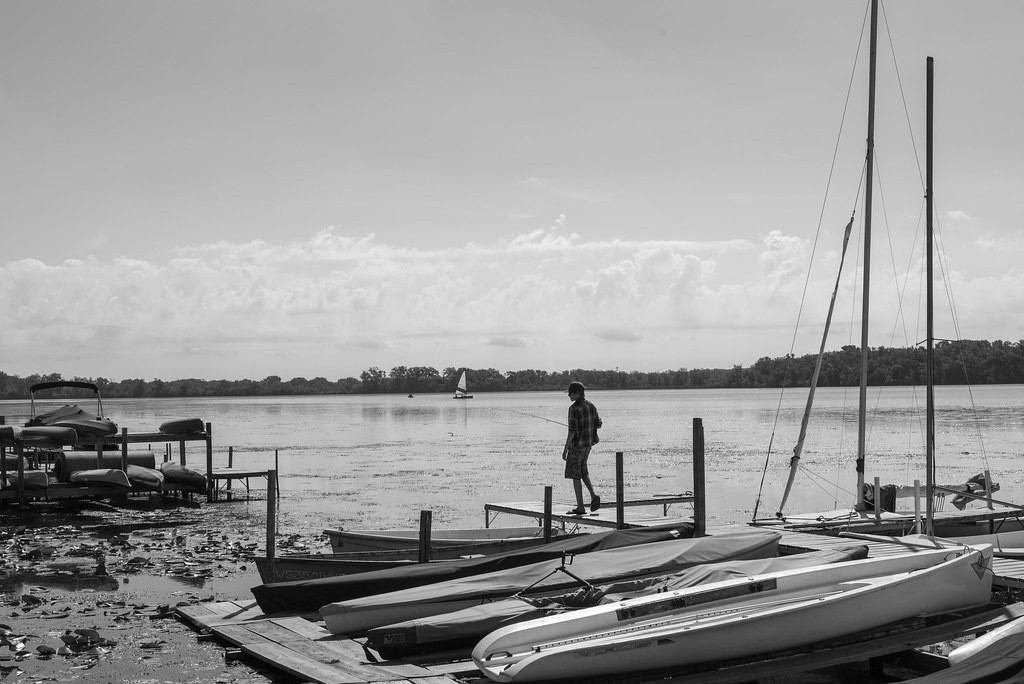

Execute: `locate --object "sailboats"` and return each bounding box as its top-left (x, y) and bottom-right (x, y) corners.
top-left (745, 1), bottom-right (1024, 535)
top-left (453, 371), bottom-right (473, 398)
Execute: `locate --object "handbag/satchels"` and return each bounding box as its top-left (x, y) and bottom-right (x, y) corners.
top-left (593, 415), bottom-right (599, 444)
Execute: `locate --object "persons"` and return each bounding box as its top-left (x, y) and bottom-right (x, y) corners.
top-left (562, 382), bottom-right (602, 514)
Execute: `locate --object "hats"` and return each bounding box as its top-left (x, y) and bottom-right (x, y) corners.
top-left (565, 382), bottom-right (585, 393)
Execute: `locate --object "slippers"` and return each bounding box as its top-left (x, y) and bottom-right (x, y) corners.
top-left (591, 496), bottom-right (600, 511)
top-left (567, 509), bottom-right (586, 514)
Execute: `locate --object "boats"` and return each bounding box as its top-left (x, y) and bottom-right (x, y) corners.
top-left (893, 614), bottom-right (1024, 683)
top-left (317, 531), bottom-right (783, 636)
top-left (472, 539), bottom-right (995, 684)
top-left (366, 544), bottom-right (870, 656)
top-left (0, 381), bottom-right (208, 496)
top-left (248, 521), bottom-right (698, 615)
